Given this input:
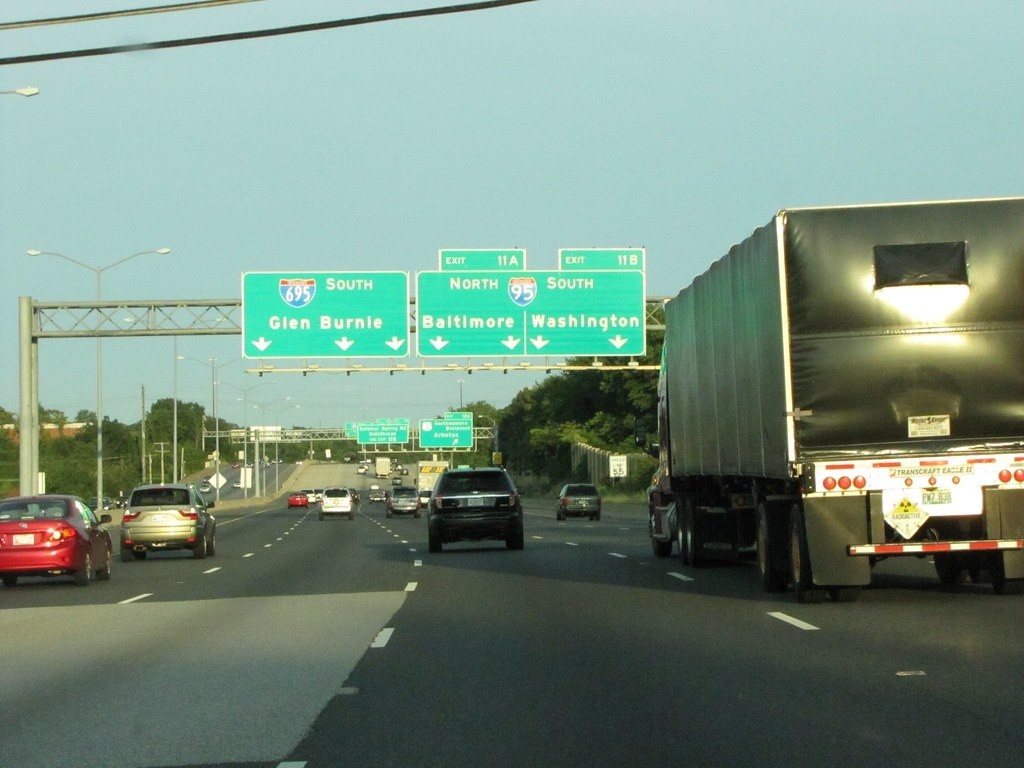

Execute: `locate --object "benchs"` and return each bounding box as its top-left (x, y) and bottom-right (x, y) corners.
top-left (141, 497), bottom-right (185, 504)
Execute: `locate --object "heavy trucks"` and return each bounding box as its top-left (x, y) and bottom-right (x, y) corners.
top-left (635, 196), bottom-right (1024, 606)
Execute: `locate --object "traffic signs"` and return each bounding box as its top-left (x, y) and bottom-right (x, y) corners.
top-left (413, 245), bottom-right (648, 358)
top-left (418, 411), bottom-right (474, 448)
top-left (238, 268), bottom-right (411, 361)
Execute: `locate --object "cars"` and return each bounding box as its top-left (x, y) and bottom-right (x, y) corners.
top-left (87, 496), bottom-right (115, 511)
top-left (113, 496), bottom-right (129, 509)
top-left (198, 454), bottom-right (284, 495)
top-left (0, 493), bottom-right (113, 587)
top-left (287, 455), bottom-right (434, 509)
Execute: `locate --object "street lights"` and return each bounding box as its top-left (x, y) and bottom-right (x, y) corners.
top-left (178, 355), bottom-right (242, 502)
top-left (237, 396), bottom-right (292, 499)
top-left (25, 246), bottom-right (172, 510)
top-left (478, 415), bottom-right (497, 452)
top-left (252, 405), bottom-right (302, 493)
top-left (456, 379), bottom-right (465, 412)
top-left (213, 380), bottom-right (279, 500)
top-left (122, 315), bottom-right (228, 484)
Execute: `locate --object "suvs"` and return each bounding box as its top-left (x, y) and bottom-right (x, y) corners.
top-left (120, 482), bottom-right (218, 560)
top-left (385, 485), bottom-right (423, 519)
top-left (419, 465), bottom-right (525, 554)
top-left (315, 486), bottom-right (359, 521)
top-left (555, 484), bottom-right (602, 522)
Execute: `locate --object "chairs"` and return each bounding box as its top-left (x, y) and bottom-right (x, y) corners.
top-left (48, 507), bottom-right (65, 517)
top-left (26, 510), bottom-right (43, 517)
top-left (1, 510), bottom-right (20, 519)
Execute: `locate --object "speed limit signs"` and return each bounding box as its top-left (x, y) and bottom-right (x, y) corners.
top-left (608, 454), bottom-right (628, 478)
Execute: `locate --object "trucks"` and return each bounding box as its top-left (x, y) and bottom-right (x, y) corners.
top-left (412, 461), bottom-right (449, 492)
